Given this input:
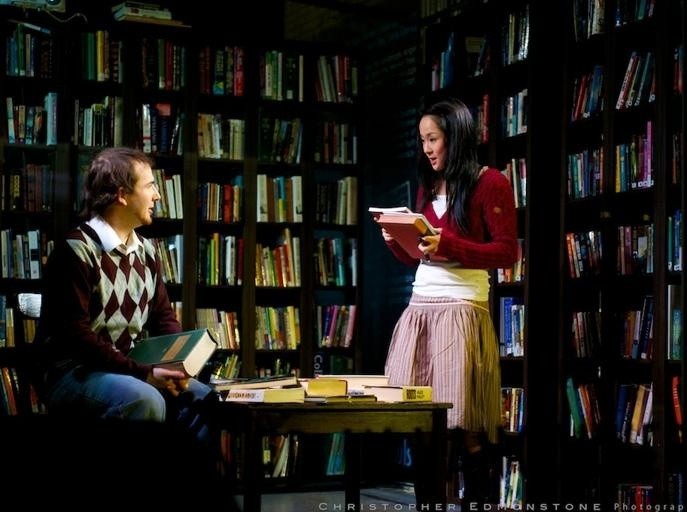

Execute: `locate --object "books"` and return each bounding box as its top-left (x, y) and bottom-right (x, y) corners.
top-left (0, 0), bottom-right (684, 512)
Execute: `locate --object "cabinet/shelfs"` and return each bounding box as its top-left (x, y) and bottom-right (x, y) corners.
top-left (0, 0), bottom-right (687, 512)
